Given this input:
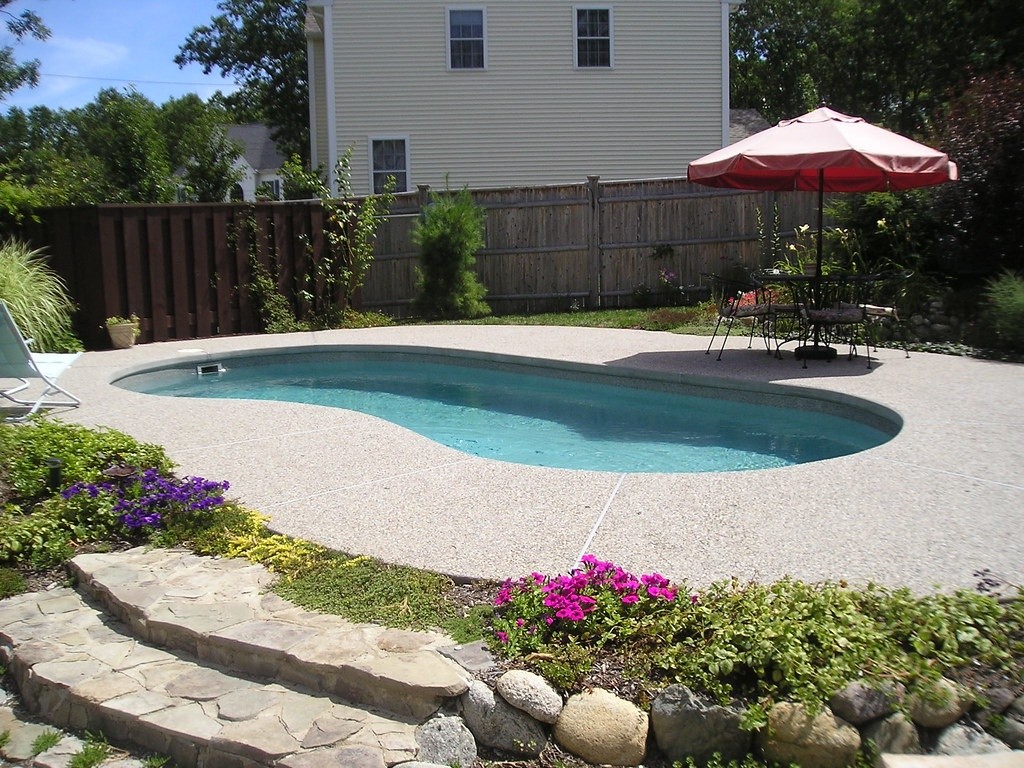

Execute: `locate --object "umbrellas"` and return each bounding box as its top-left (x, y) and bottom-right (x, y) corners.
top-left (686, 107), bottom-right (957, 361)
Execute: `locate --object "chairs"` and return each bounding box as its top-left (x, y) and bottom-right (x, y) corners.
top-left (709, 268), bottom-right (916, 372)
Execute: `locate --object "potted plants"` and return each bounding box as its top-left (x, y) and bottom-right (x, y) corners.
top-left (105, 314), bottom-right (140, 349)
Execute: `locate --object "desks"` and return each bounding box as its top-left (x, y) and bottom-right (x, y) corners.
top-left (759, 272), bottom-right (888, 361)
